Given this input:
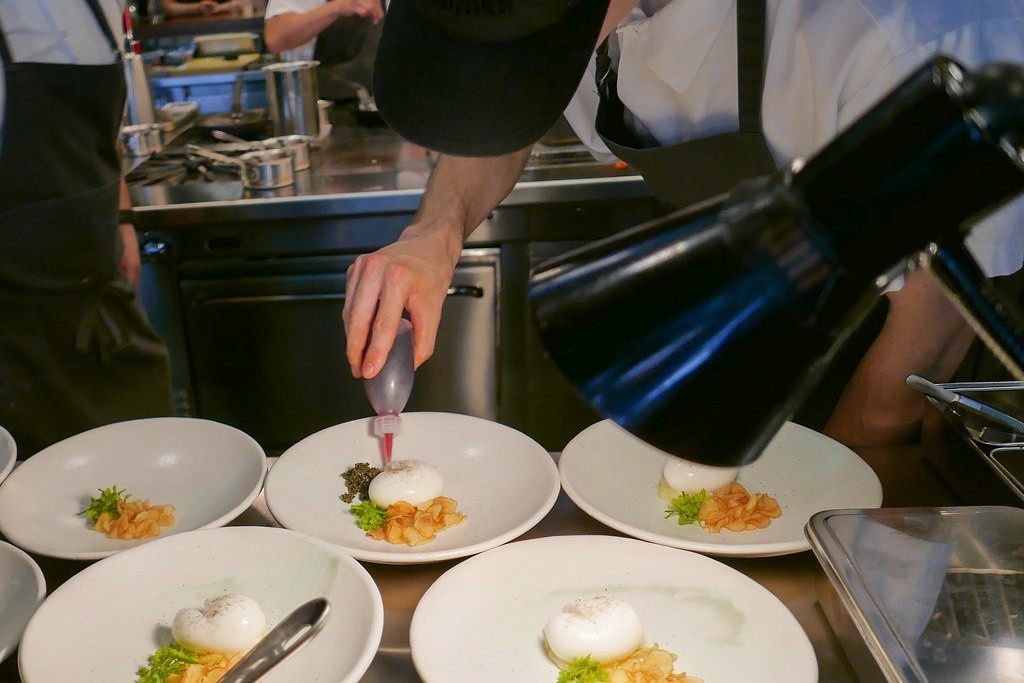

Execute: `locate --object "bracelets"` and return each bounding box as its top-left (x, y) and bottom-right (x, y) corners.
top-left (118, 209), bottom-right (137, 225)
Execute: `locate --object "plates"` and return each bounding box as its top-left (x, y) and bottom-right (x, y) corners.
top-left (408, 535), bottom-right (819, 683)
top-left (17, 525), bottom-right (384, 683)
top-left (0, 426), bottom-right (18, 485)
top-left (559, 417), bottom-right (883, 558)
top-left (0, 540), bottom-right (47, 665)
top-left (0, 418), bottom-right (268, 561)
top-left (265, 411), bottom-right (559, 566)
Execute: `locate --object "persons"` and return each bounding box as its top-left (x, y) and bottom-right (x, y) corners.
top-left (159, 0), bottom-right (250, 21)
top-left (342, 0), bottom-right (1024, 449)
top-left (264, 0), bottom-right (389, 99)
top-left (0, 0), bottom-right (174, 464)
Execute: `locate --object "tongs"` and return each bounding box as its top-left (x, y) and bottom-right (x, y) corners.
top-left (906, 374), bottom-right (1024, 445)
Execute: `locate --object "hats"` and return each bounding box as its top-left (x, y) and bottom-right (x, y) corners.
top-left (372, 0), bottom-right (612, 160)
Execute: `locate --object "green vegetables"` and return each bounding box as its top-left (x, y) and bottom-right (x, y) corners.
top-left (350, 498), bottom-right (391, 531)
top-left (664, 487), bottom-right (707, 528)
top-left (554, 653), bottom-right (614, 683)
top-left (135, 641), bottom-right (199, 683)
top-left (75, 485), bottom-right (132, 523)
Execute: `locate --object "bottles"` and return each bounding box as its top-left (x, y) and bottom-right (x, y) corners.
top-left (363, 318), bottom-right (414, 462)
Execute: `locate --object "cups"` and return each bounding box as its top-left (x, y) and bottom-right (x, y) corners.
top-left (263, 59), bottom-right (320, 137)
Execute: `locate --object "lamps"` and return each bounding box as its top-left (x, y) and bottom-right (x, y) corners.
top-left (526, 56), bottom-right (1023, 467)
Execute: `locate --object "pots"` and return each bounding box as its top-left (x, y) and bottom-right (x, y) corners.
top-left (194, 71), bottom-right (269, 137)
top-left (185, 131), bottom-right (313, 191)
